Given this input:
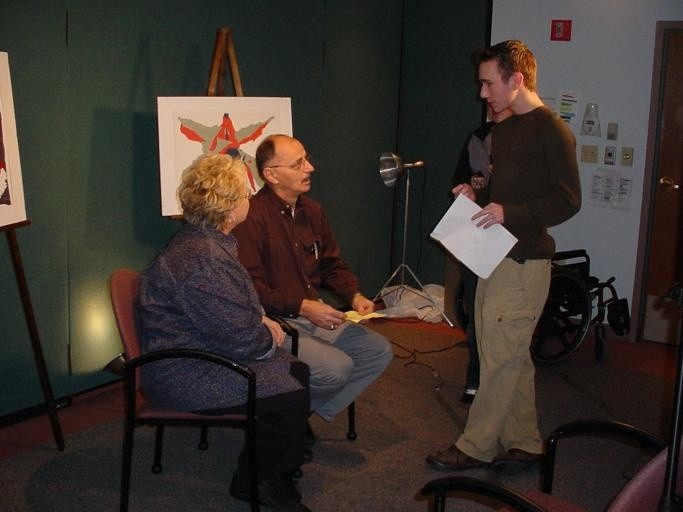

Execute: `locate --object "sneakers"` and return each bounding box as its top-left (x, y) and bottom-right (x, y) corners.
top-left (257, 478), bottom-right (311, 512)
top-left (459, 389), bottom-right (478, 409)
top-left (229, 471), bottom-right (252, 502)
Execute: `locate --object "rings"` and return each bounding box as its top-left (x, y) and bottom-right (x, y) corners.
top-left (282, 332), bottom-right (287, 336)
top-left (329, 324), bottom-right (334, 330)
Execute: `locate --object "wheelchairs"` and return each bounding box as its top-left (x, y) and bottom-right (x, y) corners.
top-left (453, 249), bottom-right (633, 362)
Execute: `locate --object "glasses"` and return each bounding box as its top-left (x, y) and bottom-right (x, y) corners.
top-left (240, 192), bottom-right (252, 199)
top-left (269, 156), bottom-right (310, 168)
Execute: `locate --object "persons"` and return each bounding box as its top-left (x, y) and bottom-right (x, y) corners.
top-left (426, 39), bottom-right (585, 471)
top-left (452, 101), bottom-right (514, 407)
top-left (137, 151), bottom-right (315, 511)
top-left (230, 134), bottom-right (396, 465)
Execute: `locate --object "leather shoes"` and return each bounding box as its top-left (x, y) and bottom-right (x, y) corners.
top-left (425, 444), bottom-right (490, 471)
top-left (490, 449), bottom-right (538, 474)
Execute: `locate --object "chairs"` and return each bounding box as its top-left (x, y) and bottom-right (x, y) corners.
top-left (421, 374), bottom-right (682, 509)
top-left (106, 267), bottom-right (301, 510)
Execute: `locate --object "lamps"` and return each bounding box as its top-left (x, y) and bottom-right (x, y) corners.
top-left (369, 151), bottom-right (454, 328)
